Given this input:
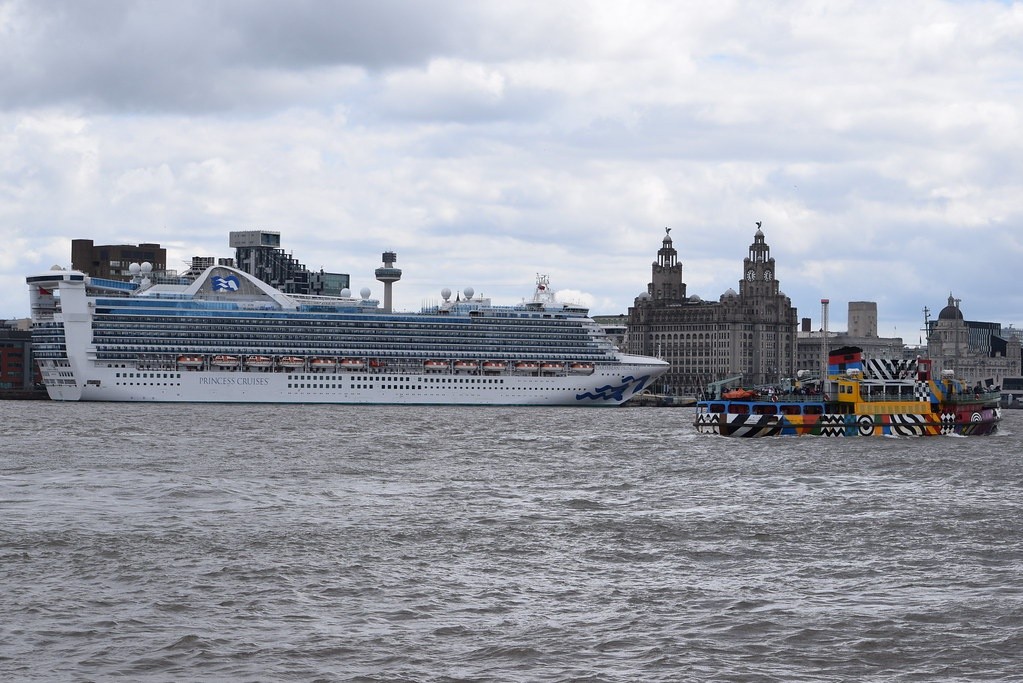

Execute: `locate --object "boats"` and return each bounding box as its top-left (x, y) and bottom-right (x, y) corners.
top-left (245, 355), bottom-right (275, 367)
top-left (454, 359), bottom-right (478, 371)
top-left (311, 357), bottom-right (335, 369)
top-left (482, 359), bottom-right (505, 372)
top-left (540, 363), bottom-right (563, 372)
top-left (515, 360), bottom-right (538, 372)
top-left (424, 360), bottom-right (449, 373)
top-left (211, 355), bottom-right (239, 367)
top-left (569, 363), bottom-right (592, 373)
top-left (694, 297), bottom-right (1004, 438)
top-left (177, 355), bottom-right (203, 367)
top-left (340, 357), bottom-right (363, 369)
top-left (278, 355), bottom-right (305, 367)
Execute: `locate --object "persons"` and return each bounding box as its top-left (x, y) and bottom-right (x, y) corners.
top-left (700, 384), bottom-right (1002, 402)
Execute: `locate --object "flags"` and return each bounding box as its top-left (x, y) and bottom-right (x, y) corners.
top-left (38, 285), bottom-right (52, 295)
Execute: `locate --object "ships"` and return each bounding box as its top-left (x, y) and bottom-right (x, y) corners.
top-left (24, 228), bottom-right (672, 409)
